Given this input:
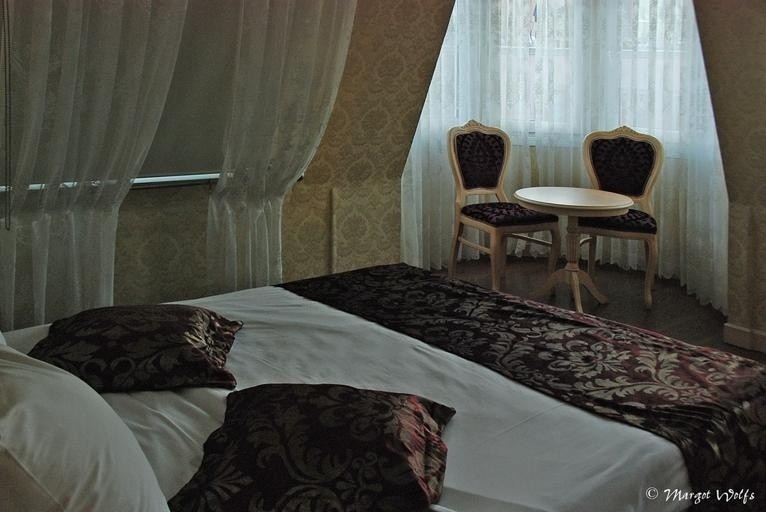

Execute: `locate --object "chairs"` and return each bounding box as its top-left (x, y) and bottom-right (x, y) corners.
top-left (580, 127), bottom-right (664, 307)
top-left (449, 121), bottom-right (561, 293)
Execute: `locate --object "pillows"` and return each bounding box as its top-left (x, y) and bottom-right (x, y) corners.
top-left (26, 305), bottom-right (242, 395)
top-left (169, 384), bottom-right (456, 512)
top-left (0, 335), bottom-right (172, 512)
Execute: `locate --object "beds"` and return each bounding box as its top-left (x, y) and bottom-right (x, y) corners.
top-left (0, 264), bottom-right (765, 512)
